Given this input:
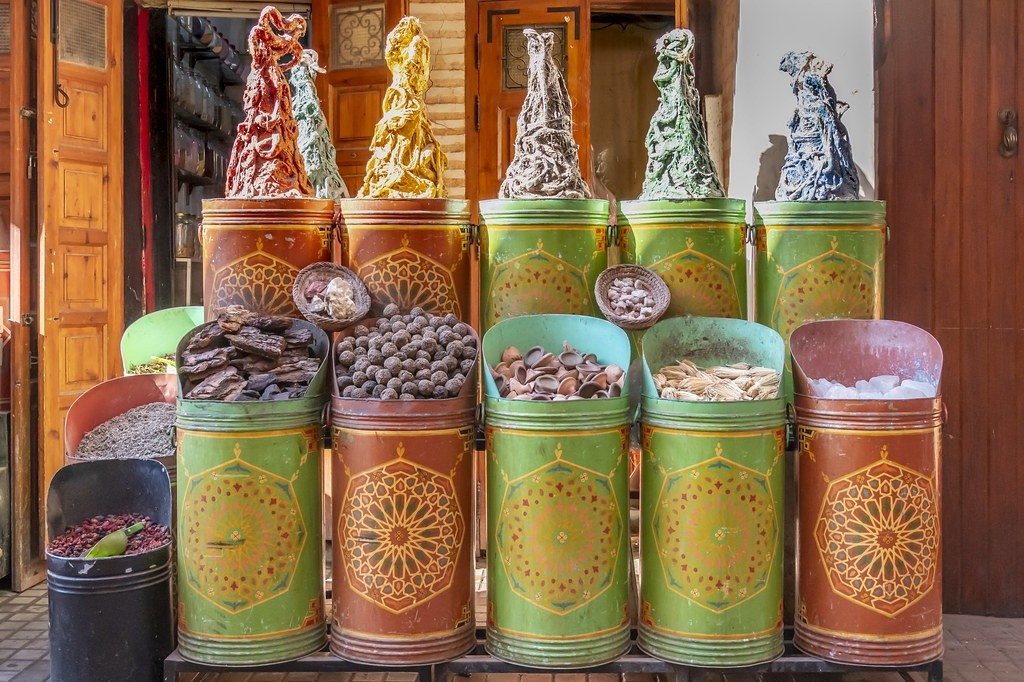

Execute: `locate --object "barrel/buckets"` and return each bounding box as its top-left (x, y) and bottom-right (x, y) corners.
top-left (44, 196), bottom-right (952, 682)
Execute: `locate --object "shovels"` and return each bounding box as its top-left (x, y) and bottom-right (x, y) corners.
top-left (151, 355), bottom-right (176, 374)
top-left (78, 523), bottom-right (146, 560)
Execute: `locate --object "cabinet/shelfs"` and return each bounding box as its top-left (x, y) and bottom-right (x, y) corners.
top-left (167, 16), bottom-right (247, 306)
